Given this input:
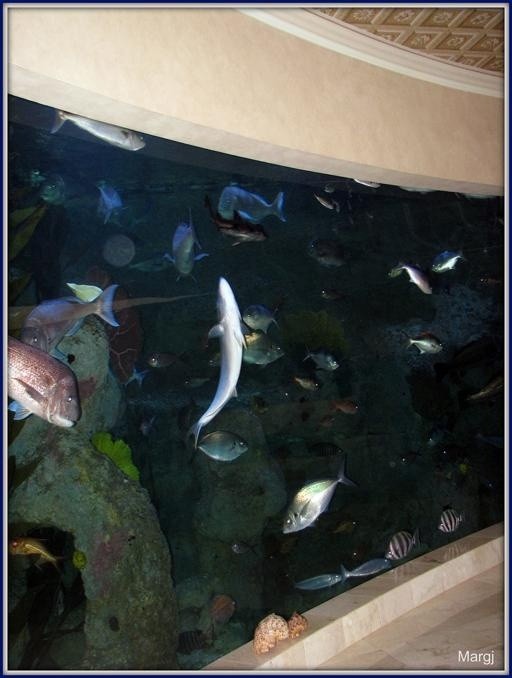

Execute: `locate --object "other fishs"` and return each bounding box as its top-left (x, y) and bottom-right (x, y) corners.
top-left (8, 163), bottom-right (505, 591)
